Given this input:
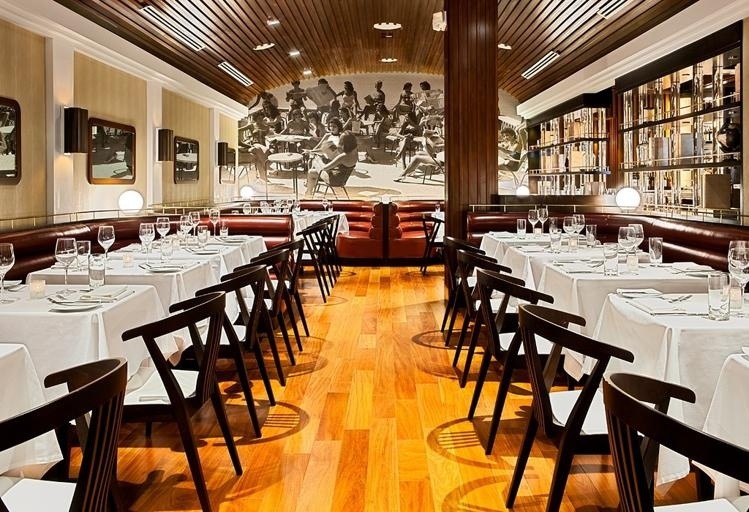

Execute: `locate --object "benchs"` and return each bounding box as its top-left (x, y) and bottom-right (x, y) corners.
top-left (382, 198), bottom-right (446, 264)
top-left (299, 197), bottom-right (384, 265)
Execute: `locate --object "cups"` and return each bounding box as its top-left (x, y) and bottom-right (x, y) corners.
top-left (122, 253), bottom-right (134, 268)
top-left (160, 238), bottom-right (174, 263)
top-left (585, 224), bottom-right (598, 248)
top-left (534, 227), bottom-right (542, 238)
top-left (648, 237), bottom-right (663, 268)
top-left (198, 225), bottom-right (208, 248)
top-left (232, 210), bottom-right (239, 215)
top-left (75, 240), bottom-right (91, 272)
top-left (626, 256), bottom-right (639, 273)
top-left (722, 286), bottom-right (743, 314)
top-left (603, 242), bottom-right (619, 276)
top-left (219, 221), bottom-right (229, 240)
top-left (516, 219), bottom-right (526, 239)
top-left (329, 204), bottom-right (334, 213)
top-left (30, 279), bottom-right (46, 299)
top-left (549, 228), bottom-right (562, 253)
top-left (707, 271), bottom-right (731, 321)
top-left (88, 253), bottom-right (106, 292)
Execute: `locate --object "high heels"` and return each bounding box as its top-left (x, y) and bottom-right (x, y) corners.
top-left (368, 156), bottom-right (378, 164)
top-left (393, 176), bottom-right (405, 182)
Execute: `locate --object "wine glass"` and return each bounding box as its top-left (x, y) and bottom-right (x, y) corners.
top-left (180, 215), bottom-right (193, 250)
top-left (571, 214), bottom-right (586, 249)
top-left (562, 217), bottom-right (578, 253)
top-left (728, 247), bottom-right (749, 317)
top-left (97, 225), bottom-right (113, 270)
top-left (156, 217), bottom-right (171, 240)
top-left (242, 200), bottom-right (302, 215)
top-left (617, 226), bottom-right (637, 257)
top-left (189, 211), bottom-right (201, 241)
top-left (0, 243), bottom-right (16, 303)
top-left (628, 223), bottom-right (645, 247)
top-left (322, 199), bottom-right (328, 212)
top-left (728, 240), bottom-right (748, 274)
top-left (138, 223), bottom-right (156, 265)
top-left (528, 210), bottom-right (540, 235)
top-left (55, 238), bottom-right (78, 295)
top-left (537, 209), bottom-right (549, 235)
top-left (209, 209), bottom-right (220, 241)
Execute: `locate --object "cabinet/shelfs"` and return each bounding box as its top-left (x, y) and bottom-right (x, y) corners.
top-left (524, 93), bottom-right (615, 199)
top-left (613, 18), bottom-right (748, 217)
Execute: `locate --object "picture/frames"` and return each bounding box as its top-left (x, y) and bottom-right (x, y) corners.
top-left (218, 147), bottom-right (237, 187)
top-left (0, 95), bottom-right (23, 186)
top-left (173, 135), bottom-right (201, 186)
top-left (85, 116), bottom-right (137, 186)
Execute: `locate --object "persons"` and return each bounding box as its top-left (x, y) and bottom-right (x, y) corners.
top-left (238, 77), bottom-right (527, 202)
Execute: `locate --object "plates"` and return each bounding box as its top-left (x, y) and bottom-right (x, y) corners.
top-left (2, 279), bottom-right (23, 288)
top-left (149, 266), bottom-right (181, 273)
top-left (51, 300), bottom-right (101, 312)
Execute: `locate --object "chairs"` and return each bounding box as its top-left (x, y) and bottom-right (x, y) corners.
top-left (237, 102), bottom-right (529, 198)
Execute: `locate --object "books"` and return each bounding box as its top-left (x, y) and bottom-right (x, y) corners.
top-left (536, 44), bottom-right (742, 216)
